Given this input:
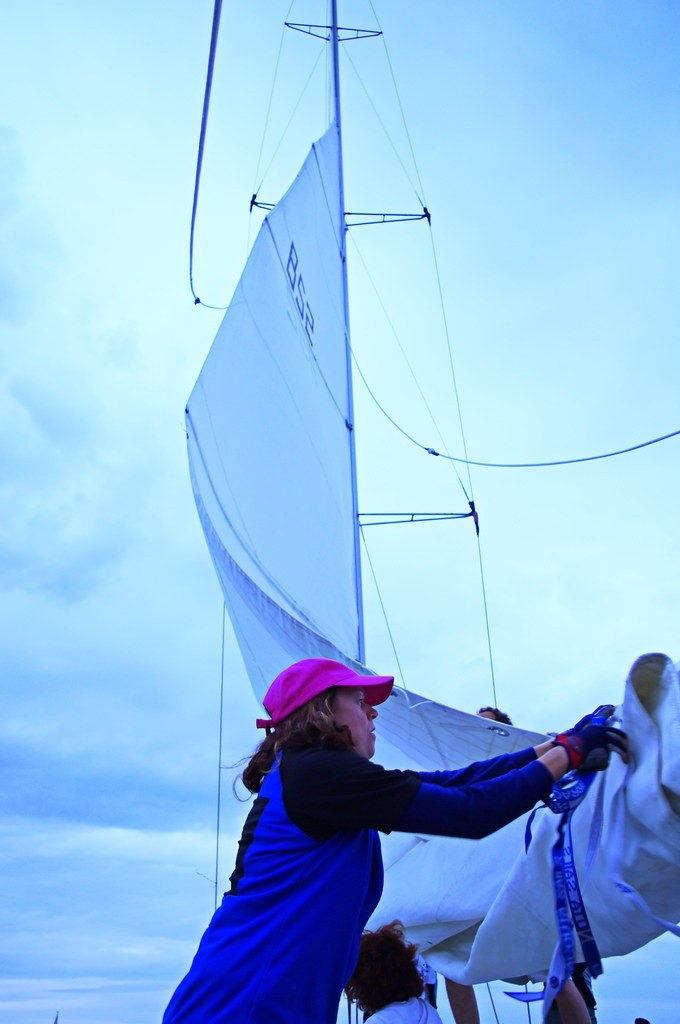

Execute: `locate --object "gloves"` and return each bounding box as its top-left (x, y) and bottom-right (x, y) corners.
top-left (559, 704), bottom-right (614, 734)
top-left (552, 723), bottom-right (629, 774)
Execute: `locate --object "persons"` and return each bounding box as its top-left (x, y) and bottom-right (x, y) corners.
top-left (446, 706), bottom-right (593, 1024)
top-left (163, 659), bottom-right (629, 1024)
top-left (346, 921), bottom-right (444, 1024)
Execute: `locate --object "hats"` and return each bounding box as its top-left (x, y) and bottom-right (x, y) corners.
top-left (256, 657), bottom-right (394, 729)
